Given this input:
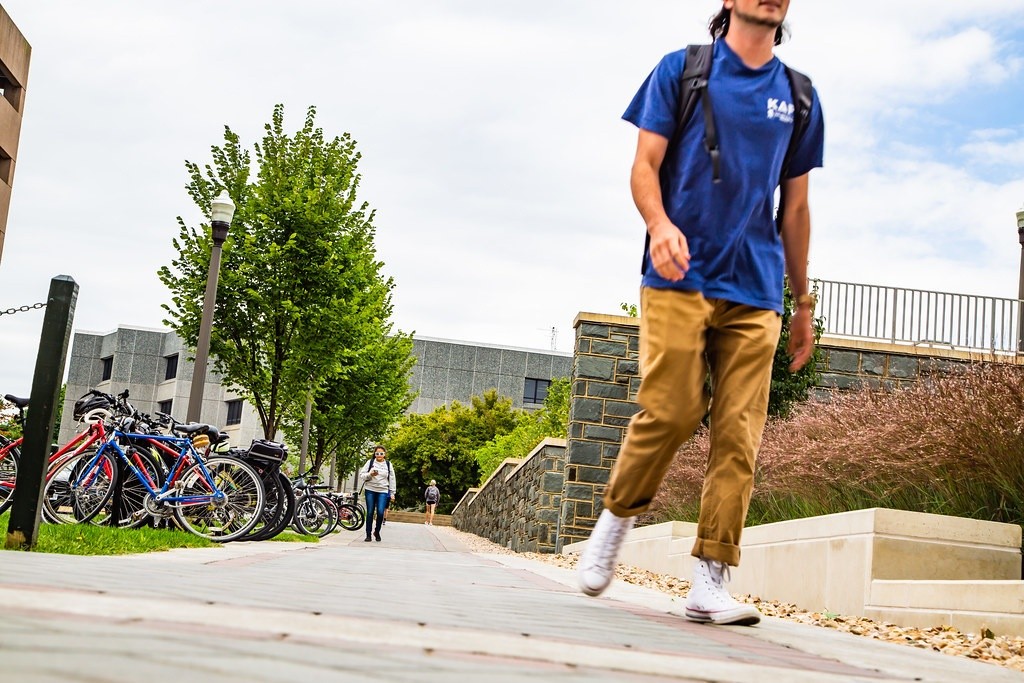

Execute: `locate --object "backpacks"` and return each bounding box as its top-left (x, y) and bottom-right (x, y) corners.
top-left (428, 492), bottom-right (436, 499)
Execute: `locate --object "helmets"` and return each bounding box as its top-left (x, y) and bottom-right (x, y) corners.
top-left (74, 397), bottom-right (110, 422)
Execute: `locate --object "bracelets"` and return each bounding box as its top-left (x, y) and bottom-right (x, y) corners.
top-left (797, 295), bottom-right (813, 306)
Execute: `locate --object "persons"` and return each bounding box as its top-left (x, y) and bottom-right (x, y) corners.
top-left (360, 446), bottom-right (396, 542)
top-left (580, 0), bottom-right (823, 624)
top-left (425, 480), bottom-right (440, 526)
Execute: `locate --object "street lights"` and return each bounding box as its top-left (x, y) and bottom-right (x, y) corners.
top-left (183, 189), bottom-right (236, 425)
top-left (1015, 200), bottom-right (1024, 357)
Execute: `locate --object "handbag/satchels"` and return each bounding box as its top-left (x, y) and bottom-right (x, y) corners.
top-left (248, 438), bottom-right (287, 465)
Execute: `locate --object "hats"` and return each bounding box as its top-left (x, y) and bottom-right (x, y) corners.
top-left (431, 480), bottom-right (436, 485)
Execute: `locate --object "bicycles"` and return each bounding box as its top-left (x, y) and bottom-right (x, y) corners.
top-left (0, 388), bottom-right (366, 542)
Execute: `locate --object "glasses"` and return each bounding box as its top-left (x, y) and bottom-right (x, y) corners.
top-left (375, 453), bottom-right (384, 456)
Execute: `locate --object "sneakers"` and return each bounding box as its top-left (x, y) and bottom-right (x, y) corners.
top-left (683, 559), bottom-right (762, 624)
top-left (577, 508), bottom-right (637, 598)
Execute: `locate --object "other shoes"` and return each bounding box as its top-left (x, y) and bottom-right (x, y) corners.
top-left (425, 522), bottom-right (428, 525)
top-left (373, 531), bottom-right (382, 542)
top-left (429, 523), bottom-right (432, 525)
top-left (365, 533), bottom-right (372, 541)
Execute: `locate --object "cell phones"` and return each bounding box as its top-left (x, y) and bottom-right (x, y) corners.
top-left (374, 470), bottom-right (378, 472)
